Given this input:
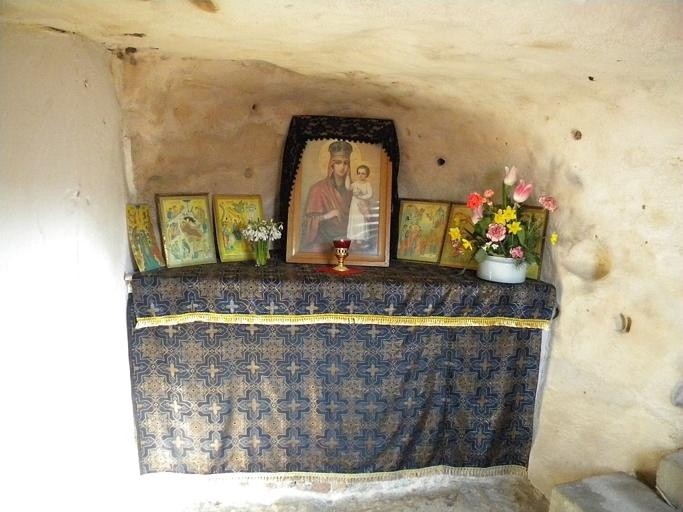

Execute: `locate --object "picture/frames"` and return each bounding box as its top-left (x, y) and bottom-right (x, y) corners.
top-left (123, 259), bottom-right (559, 492)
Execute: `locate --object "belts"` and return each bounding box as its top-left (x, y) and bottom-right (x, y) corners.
top-left (332, 239), bottom-right (351, 272)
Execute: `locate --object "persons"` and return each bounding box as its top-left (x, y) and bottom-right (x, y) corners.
top-left (302, 141), bottom-right (354, 252)
top-left (344, 165), bottom-right (373, 250)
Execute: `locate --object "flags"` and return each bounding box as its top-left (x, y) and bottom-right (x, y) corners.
top-left (254, 238), bottom-right (269, 266)
top-left (477, 256), bottom-right (527, 284)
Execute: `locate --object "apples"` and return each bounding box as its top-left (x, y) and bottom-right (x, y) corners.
top-left (333, 240), bottom-right (351, 248)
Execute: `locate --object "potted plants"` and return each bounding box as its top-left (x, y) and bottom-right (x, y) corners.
top-left (286, 114), bottom-right (394, 268)
top-left (212, 193), bottom-right (271, 263)
top-left (438, 201), bottom-right (502, 271)
top-left (396, 197), bottom-right (451, 265)
top-left (153, 192), bottom-right (218, 269)
top-left (516, 206), bottom-right (549, 281)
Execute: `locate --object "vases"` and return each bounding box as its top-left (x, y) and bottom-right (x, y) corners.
top-left (242, 217), bottom-right (283, 266)
top-left (448, 165), bottom-right (560, 275)
